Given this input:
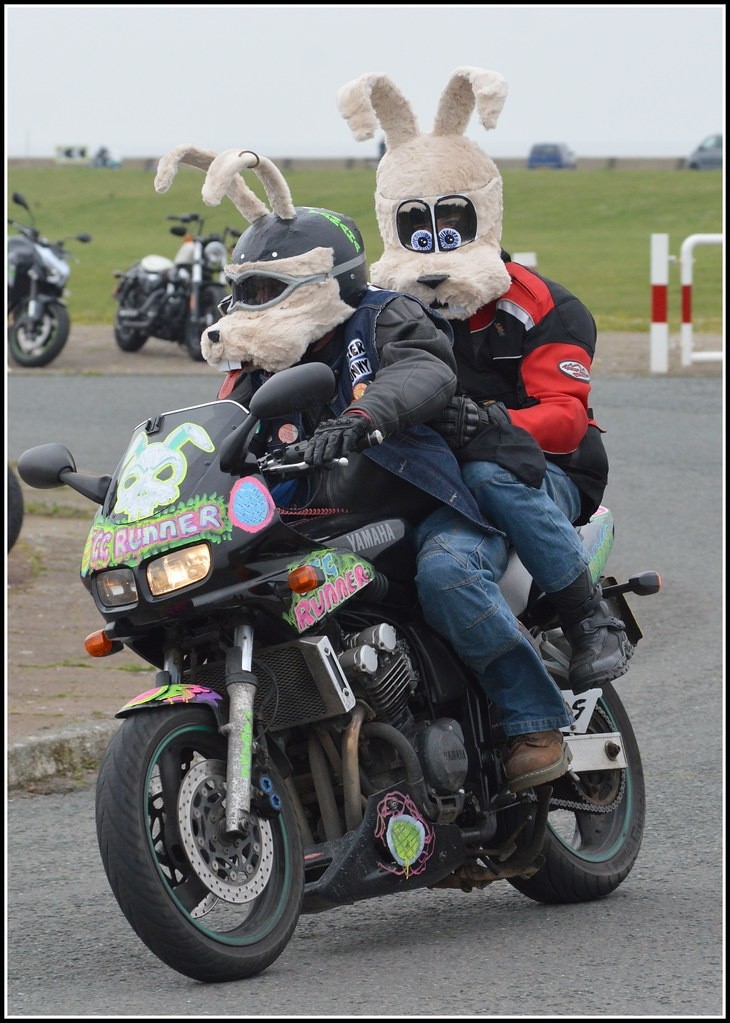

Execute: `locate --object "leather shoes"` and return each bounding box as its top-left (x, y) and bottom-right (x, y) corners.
top-left (502, 728), bottom-right (573, 792)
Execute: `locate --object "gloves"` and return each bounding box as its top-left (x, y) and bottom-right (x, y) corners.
top-left (428, 396), bottom-right (512, 450)
top-left (304, 413), bottom-right (372, 471)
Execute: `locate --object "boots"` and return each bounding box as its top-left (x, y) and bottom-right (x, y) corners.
top-left (547, 571), bottom-right (635, 696)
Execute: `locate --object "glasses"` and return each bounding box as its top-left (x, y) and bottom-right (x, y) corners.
top-left (224, 275), bottom-right (300, 315)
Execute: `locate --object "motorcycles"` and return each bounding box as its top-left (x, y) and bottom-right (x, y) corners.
top-left (8, 192), bottom-right (92, 368)
top-left (112, 213), bottom-right (243, 361)
top-left (17, 362), bottom-right (662, 982)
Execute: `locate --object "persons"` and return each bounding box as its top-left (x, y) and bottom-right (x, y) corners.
top-left (369, 136), bottom-right (634, 692)
top-left (198, 208), bottom-right (573, 793)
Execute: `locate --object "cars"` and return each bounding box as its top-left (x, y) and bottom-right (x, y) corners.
top-left (529, 140), bottom-right (577, 169)
top-left (675, 132), bottom-right (723, 170)
